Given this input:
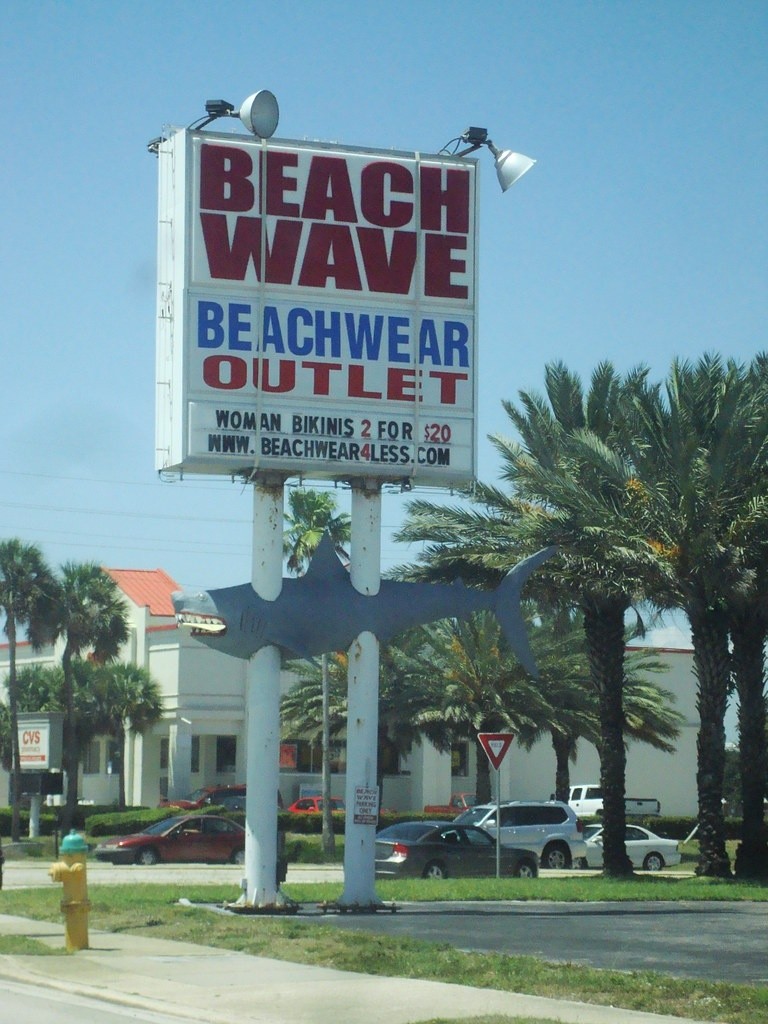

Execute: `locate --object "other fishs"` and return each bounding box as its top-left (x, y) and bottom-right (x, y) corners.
top-left (172, 524), bottom-right (561, 681)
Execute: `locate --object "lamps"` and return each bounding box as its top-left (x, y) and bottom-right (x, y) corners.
top-left (437, 128), bottom-right (538, 193)
top-left (191, 89), bottom-right (280, 139)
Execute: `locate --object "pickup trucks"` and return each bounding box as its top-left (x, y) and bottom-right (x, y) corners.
top-left (424, 791), bottom-right (495, 813)
top-left (555, 786), bottom-right (663, 817)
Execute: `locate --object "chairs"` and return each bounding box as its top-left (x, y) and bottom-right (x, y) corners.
top-left (445, 833), bottom-right (458, 842)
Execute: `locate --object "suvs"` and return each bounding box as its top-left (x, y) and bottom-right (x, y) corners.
top-left (453, 800), bottom-right (589, 870)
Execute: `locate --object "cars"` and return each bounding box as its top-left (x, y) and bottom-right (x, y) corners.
top-left (93, 816), bottom-right (244, 867)
top-left (288, 797), bottom-right (346, 814)
top-left (375, 820), bottom-right (540, 879)
top-left (161, 784), bottom-right (283, 812)
top-left (582, 824), bottom-right (682, 871)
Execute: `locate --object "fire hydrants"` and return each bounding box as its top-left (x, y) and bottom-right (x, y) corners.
top-left (48, 829), bottom-right (92, 953)
